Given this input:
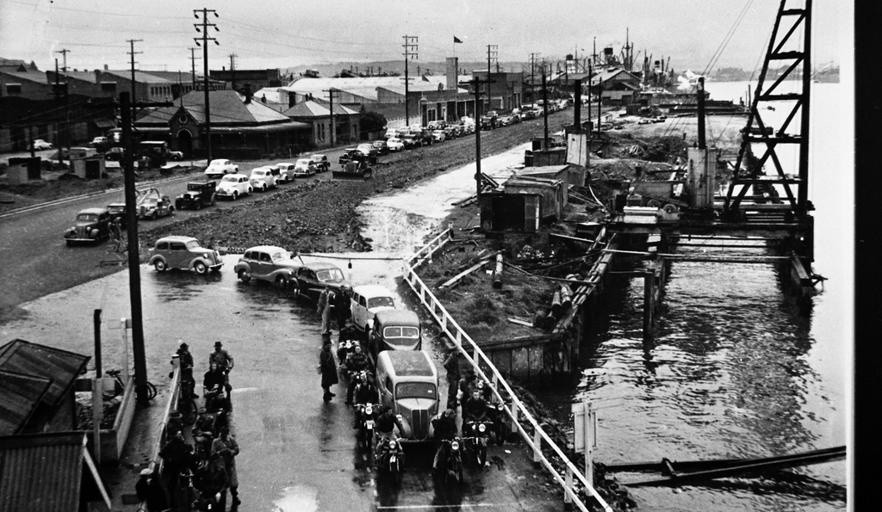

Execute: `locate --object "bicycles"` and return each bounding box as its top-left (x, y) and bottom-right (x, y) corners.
top-left (104, 367), bottom-right (230, 512)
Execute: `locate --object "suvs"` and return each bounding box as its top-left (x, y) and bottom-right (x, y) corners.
top-left (363, 309), bottom-right (434, 360)
top-left (341, 283), bottom-right (397, 336)
top-left (231, 245), bottom-right (302, 291)
top-left (370, 349), bottom-right (443, 455)
top-left (286, 259), bottom-right (352, 310)
top-left (144, 234), bottom-right (223, 276)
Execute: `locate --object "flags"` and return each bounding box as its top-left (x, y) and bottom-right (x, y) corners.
top-left (454, 36), bottom-right (463, 43)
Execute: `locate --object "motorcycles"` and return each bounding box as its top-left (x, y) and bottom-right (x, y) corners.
top-left (436, 402), bottom-right (506, 493)
top-left (335, 342), bottom-right (401, 492)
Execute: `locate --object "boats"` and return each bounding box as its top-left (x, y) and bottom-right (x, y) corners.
top-left (600, 81), bottom-right (801, 145)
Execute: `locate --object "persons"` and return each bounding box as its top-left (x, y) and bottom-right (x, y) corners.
top-left (108, 214), bottom-right (124, 253)
top-left (134, 342), bottom-right (242, 512)
top-left (317, 284), bottom-right (496, 479)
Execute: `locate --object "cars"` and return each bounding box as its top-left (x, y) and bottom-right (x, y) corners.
top-left (25, 137), bottom-right (55, 152)
